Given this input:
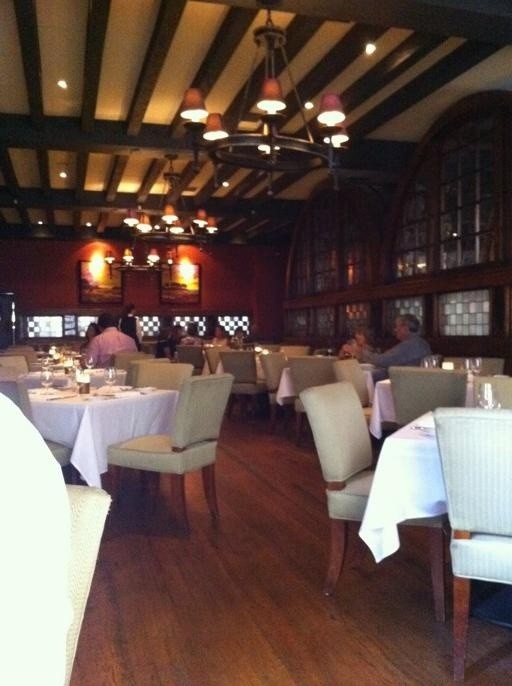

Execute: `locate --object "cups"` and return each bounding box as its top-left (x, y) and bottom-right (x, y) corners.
top-left (460, 357), bottom-right (482, 377)
top-left (419, 354), bottom-right (440, 369)
top-left (481, 383), bottom-right (493, 410)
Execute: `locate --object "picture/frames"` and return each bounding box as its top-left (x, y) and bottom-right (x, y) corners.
top-left (160, 262), bottom-right (202, 305)
top-left (78, 259), bottom-right (124, 305)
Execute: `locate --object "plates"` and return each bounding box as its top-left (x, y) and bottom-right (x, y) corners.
top-left (96, 394), bottom-right (118, 398)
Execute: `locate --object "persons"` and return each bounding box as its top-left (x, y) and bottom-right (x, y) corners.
top-left (79, 303), bottom-right (248, 369)
top-left (339, 325), bottom-right (376, 363)
top-left (361, 313), bottom-right (432, 385)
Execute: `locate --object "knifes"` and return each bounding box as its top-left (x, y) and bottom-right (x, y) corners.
top-left (46, 395), bottom-right (77, 401)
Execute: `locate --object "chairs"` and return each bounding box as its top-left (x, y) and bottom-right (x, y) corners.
top-left (49, 483), bottom-right (112, 675)
top-left (299, 357), bottom-right (511, 681)
top-left (143, 337), bottom-right (446, 433)
top-left (2, 337), bottom-right (234, 536)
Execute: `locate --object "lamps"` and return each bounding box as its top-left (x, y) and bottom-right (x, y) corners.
top-left (103, 155), bottom-right (222, 277)
top-left (179, 0), bottom-right (351, 193)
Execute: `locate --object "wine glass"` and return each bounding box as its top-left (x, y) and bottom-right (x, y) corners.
top-left (104, 366), bottom-right (118, 392)
top-left (41, 342), bottom-right (98, 400)
